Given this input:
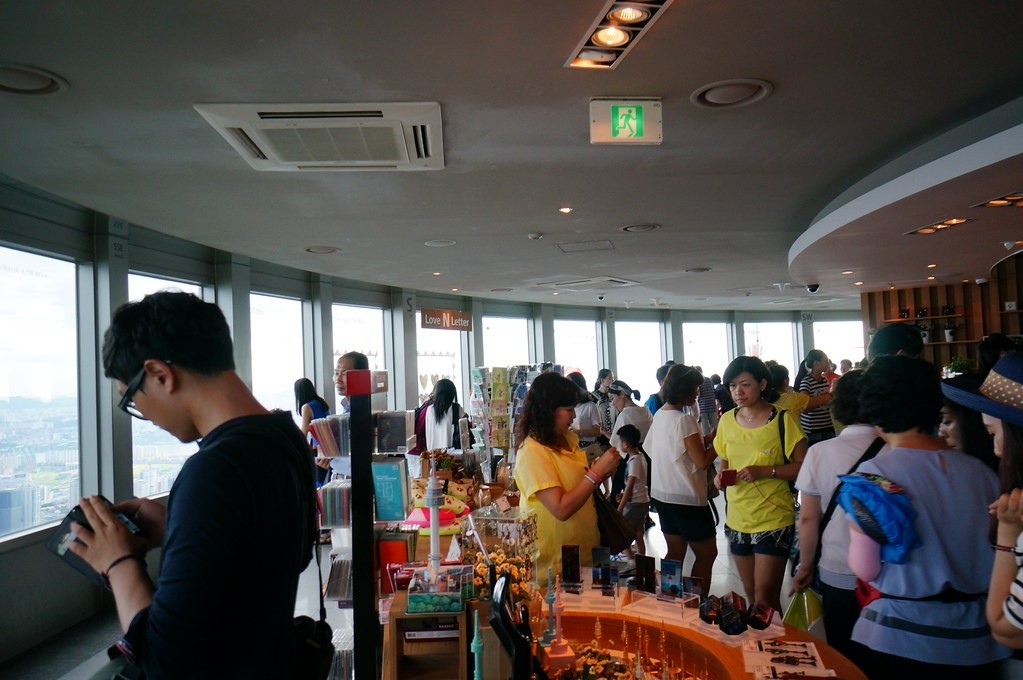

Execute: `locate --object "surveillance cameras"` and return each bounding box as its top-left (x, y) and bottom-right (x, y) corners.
top-left (976, 279), bottom-right (988, 286)
top-left (597, 294), bottom-right (606, 301)
top-left (806, 284), bottom-right (820, 293)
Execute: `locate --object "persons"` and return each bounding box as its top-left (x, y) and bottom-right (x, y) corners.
top-left (335, 352), bottom-right (368, 414)
top-left (513, 372), bottom-right (621, 591)
top-left (641, 365), bottom-right (718, 599)
top-left (849, 354), bottom-right (1001, 680)
top-left (615, 423), bottom-right (649, 556)
top-left (789, 372), bottom-right (886, 669)
top-left (712, 356), bottom-right (809, 621)
top-left (937, 332), bottom-right (1023, 680)
top-left (425, 379), bottom-right (475, 453)
top-left (294, 378), bottom-right (332, 544)
top-left (568, 325), bottom-right (924, 511)
top-left (67, 292), bottom-right (317, 680)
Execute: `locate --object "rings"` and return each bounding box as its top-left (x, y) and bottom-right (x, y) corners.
top-left (744, 478), bottom-right (747, 481)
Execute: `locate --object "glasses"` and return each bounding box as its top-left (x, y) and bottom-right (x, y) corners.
top-left (117, 360), bottom-right (172, 421)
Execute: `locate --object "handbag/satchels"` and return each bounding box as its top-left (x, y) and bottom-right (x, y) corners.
top-left (287, 615), bottom-right (334, 680)
top-left (596, 489), bottom-right (634, 556)
top-left (782, 587), bottom-right (827, 643)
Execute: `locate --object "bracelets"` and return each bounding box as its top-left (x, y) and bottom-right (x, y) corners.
top-left (586, 468), bottom-right (604, 485)
top-left (772, 465), bottom-right (776, 478)
top-left (583, 473), bottom-right (595, 486)
top-left (101, 554), bottom-right (147, 591)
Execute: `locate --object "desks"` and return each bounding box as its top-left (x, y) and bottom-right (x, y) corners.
top-left (385, 591), bottom-right (468, 680)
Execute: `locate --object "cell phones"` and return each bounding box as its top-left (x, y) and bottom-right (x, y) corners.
top-left (96, 494), bottom-right (141, 537)
top-left (720, 469), bottom-right (737, 486)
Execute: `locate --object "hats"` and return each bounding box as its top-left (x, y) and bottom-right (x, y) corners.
top-left (940, 354), bottom-right (1023, 425)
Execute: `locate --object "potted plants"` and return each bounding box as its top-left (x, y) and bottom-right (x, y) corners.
top-left (916, 320), bottom-right (931, 344)
top-left (943, 319), bottom-right (958, 342)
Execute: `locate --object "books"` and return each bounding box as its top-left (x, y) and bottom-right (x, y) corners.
top-left (307, 413), bottom-right (352, 600)
top-left (379, 560), bottom-right (474, 641)
top-left (470, 362), bottom-right (563, 449)
top-left (372, 408), bottom-right (418, 595)
top-left (326, 649), bottom-right (354, 680)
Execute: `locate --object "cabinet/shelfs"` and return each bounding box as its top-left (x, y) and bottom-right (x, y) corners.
top-left (881, 314), bottom-right (981, 345)
